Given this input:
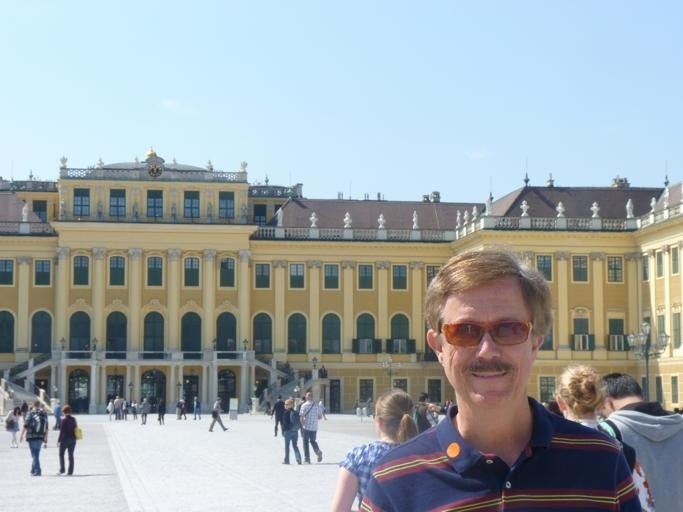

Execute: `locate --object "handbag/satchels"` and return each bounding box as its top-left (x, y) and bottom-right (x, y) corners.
top-left (73, 426), bottom-right (83, 441)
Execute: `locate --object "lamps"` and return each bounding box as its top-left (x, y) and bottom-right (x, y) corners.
top-left (242, 338), bottom-right (249, 352)
top-left (91, 334), bottom-right (100, 353)
top-left (59, 337), bottom-right (66, 350)
top-left (311, 357), bottom-right (316, 372)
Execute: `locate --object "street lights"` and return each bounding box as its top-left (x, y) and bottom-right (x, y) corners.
top-left (627, 322), bottom-right (668, 404)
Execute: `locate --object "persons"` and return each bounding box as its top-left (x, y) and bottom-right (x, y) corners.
top-left (319, 365), bottom-right (326, 378)
top-left (6, 400), bottom-right (77, 476)
top-left (208, 398), bottom-right (227, 432)
top-left (264, 386), bottom-right (328, 465)
top-left (107, 396), bottom-right (201, 425)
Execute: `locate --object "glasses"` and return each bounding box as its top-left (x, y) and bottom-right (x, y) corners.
top-left (438, 319), bottom-right (532, 347)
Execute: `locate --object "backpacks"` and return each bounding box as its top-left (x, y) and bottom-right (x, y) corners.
top-left (30, 410), bottom-right (45, 434)
top-left (287, 410), bottom-right (300, 432)
